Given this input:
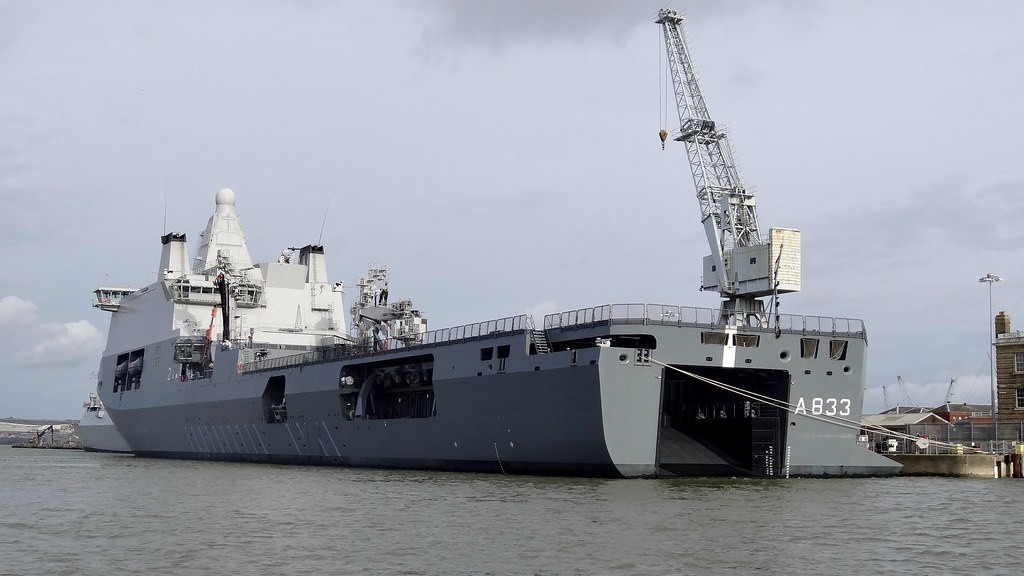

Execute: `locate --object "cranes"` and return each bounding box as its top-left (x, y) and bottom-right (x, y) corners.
top-left (881, 350), bottom-right (995, 410)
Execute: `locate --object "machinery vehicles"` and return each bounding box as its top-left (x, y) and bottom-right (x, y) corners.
top-left (30, 425), bottom-right (54, 447)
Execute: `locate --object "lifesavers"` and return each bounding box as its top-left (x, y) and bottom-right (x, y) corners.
top-left (238, 363), bottom-right (244, 370)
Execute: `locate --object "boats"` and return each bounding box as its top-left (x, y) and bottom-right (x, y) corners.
top-left (77, 372), bottom-right (133, 454)
top-left (91, 6), bottom-right (905, 482)
